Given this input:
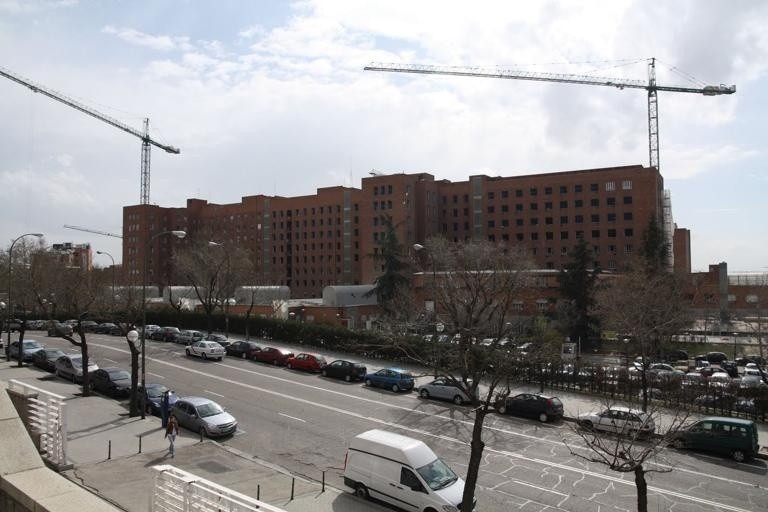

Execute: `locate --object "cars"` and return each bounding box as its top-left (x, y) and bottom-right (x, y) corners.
top-left (88, 366), bottom-right (132, 398)
top-left (170, 396), bottom-right (238, 438)
top-left (397, 332), bottom-right (542, 376)
top-left (130, 382), bottom-right (182, 416)
top-left (575, 405), bottom-right (656, 441)
top-left (2, 319), bottom-right (295, 373)
top-left (55, 353), bottom-right (100, 385)
top-left (540, 351), bottom-right (767, 422)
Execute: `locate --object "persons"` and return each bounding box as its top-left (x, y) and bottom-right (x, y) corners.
top-left (164, 416), bottom-right (178, 458)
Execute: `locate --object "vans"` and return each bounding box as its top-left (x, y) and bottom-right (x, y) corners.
top-left (418, 375), bottom-right (479, 405)
top-left (285, 351), bottom-right (327, 373)
top-left (663, 415), bottom-right (760, 462)
top-left (320, 359), bottom-right (367, 383)
top-left (495, 390), bottom-right (563, 423)
top-left (364, 368), bottom-right (414, 394)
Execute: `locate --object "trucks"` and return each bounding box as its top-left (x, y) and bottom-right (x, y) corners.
top-left (343, 429), bottom-right (477, 512)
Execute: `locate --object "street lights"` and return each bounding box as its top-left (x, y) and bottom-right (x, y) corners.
top-left (414, 243), bottom-right (445, 381)
top-left (0, 232), bottom-right (42, 363)
top-left (96, 251), bottom-right (121, 322)
top-left (732, 331), bottom-right (740, 361)
top-left (209, 242), bottom-right (237, 339)
top-left (126, 231), bottom-right (186, 421)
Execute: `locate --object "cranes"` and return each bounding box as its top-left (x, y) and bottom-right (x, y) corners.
top-left (0, 68), bottom-right (181, 205)
top-left (362, 57), bottom-right (737, 174)
